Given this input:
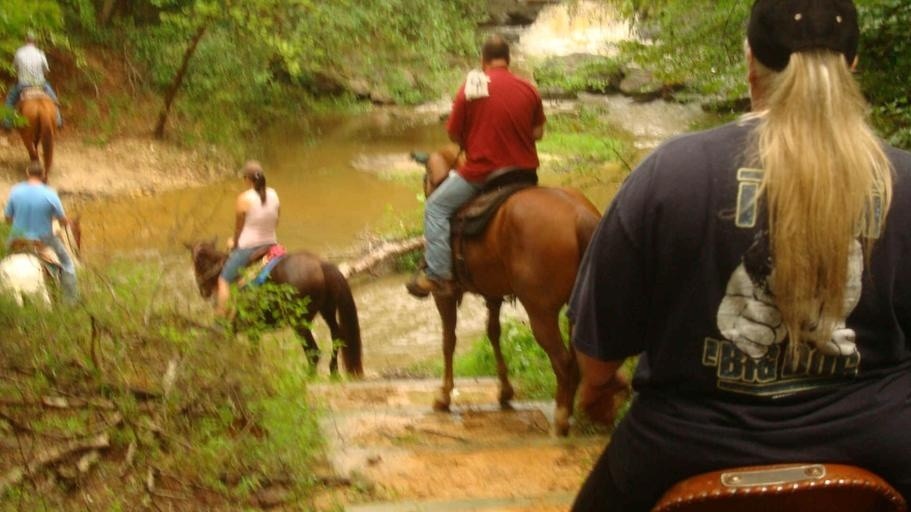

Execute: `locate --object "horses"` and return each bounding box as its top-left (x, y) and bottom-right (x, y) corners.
top-left (0, 213), bottom-right (80, 313)
top-left (409, 143), bottom-right (603, 438)
top-left (16, 86), bottom-right (58, 184)
top-left (183, 234), bottom-right (365, 381)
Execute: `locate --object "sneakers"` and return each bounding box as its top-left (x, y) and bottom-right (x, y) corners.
top-left (404, 274), bottom-right (454, 299)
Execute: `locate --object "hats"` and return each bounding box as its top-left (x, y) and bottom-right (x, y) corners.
top-left (747, 0), bottom-right (860, 73)
top-left (238, 160), bottom-right (264, 178)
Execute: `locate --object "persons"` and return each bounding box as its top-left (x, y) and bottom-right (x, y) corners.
top-left (405, 34), bottom-right (547, 307)
top-left (564, 3), bottom-right (911, 509)
top-left (209, 157), bottom-right (283, 322)
top-left (3, 162), bottom-right (78, 301)
top-left (2, 29), bottom-right (66, 136)
